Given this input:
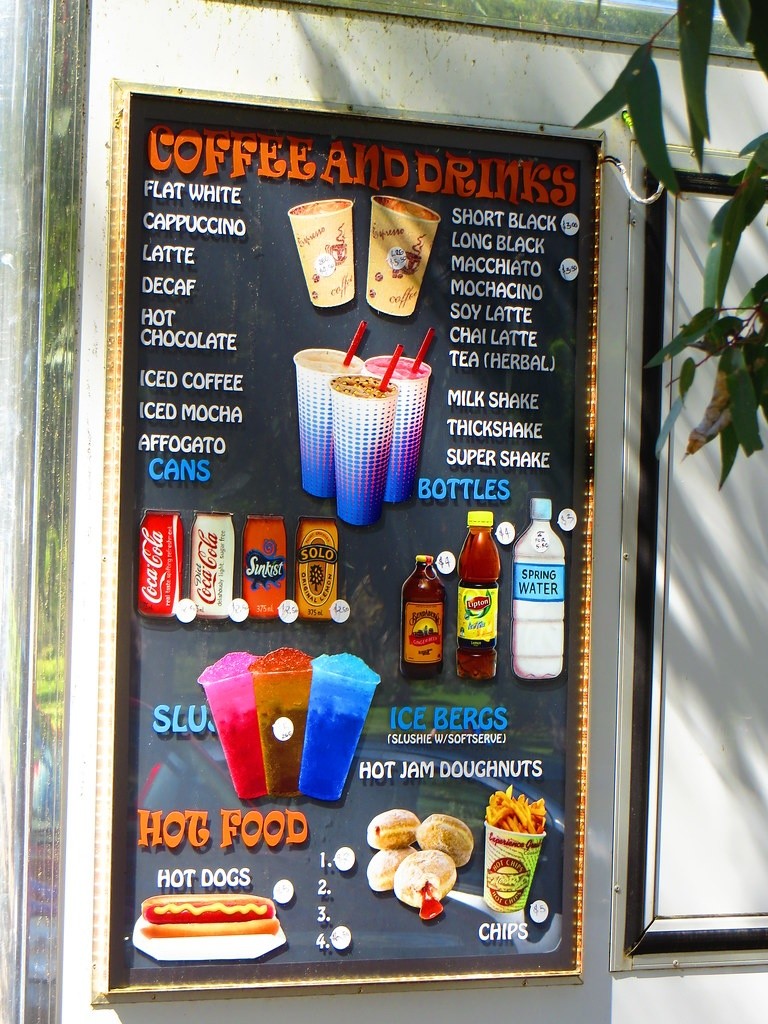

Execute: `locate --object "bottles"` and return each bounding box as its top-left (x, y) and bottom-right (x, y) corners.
top-left (399, 553), bottom-right (448, 681)
top-left (455, 509), bottom-right (502, 683)
top-left (508, 495), bottom-right (567, 682)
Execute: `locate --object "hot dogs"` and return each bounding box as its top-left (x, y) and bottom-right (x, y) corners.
top-left (140, 895), bottom-right (280, 937)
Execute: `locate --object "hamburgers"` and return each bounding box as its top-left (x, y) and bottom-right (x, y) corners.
top-left (417, 815), bottom-right (473, 866)
top-left (366, 847), bottom-right (415, 892)
top-left (367, 811), bottom-right (420, 849)
top-left (394, 850), bottom-right (457, 907)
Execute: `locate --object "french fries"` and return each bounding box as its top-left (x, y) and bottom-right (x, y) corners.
top-left (485, 783), bottom-right (548, 834)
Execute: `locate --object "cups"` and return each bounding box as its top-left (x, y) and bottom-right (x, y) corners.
top-left (298, 663), bottom-right (380, 802)
top-left (360, 355), bottom-right (434, 504)
top-left (482, 820), bottom-right (547, 914)
top-left (286, 197), bottom-right (356, 309)
top-left (292, 346), bottom-right (362, 500)
top-left (327, 375), bottom-right (401, 527)
top-left (364, 188), bottom-right (442, 318)
top-left (200, 671), bottom-right (269, 802)
top-left (249, 670), bottom-right (316, 800)
